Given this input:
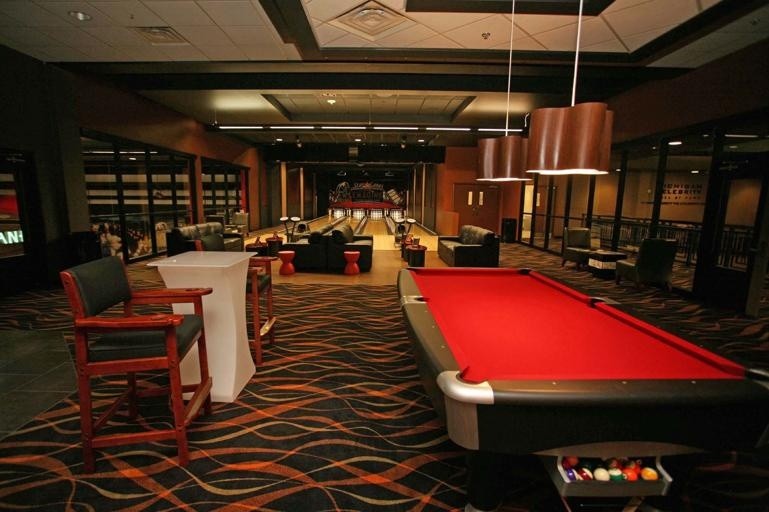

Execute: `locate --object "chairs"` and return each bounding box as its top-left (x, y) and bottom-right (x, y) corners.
top-left (58, 255), bottom-right (213, 474)
top-left (187, 232), bottom-right (277, 365)
top-left (207, 212), bottom-right (250, 237)
top-left (561, 226), bottom-right (595, 271)
top-left (615, 237), bottom-right (679, 292)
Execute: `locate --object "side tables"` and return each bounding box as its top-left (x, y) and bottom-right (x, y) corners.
top-left (277, 250), bottom-right (295, 275)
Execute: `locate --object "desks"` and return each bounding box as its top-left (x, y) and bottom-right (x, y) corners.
top-left (146, 251), bottom-right (259, 404)
top-left (397, 267), bottom-right (768, 512)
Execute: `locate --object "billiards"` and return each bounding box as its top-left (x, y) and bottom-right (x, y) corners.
top-left (561, 455), bottom-right (658, 481)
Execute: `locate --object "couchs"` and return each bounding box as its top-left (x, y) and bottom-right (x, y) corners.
top-left (165, 222), bottom-right (244, 257)
top-left (438, 224), bottom-right (500, 267)
top-left (283, 224), bottom-right (373, 274)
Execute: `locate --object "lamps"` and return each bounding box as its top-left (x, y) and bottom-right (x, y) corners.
top-left (476, 0), bottom-right (615, 182)
